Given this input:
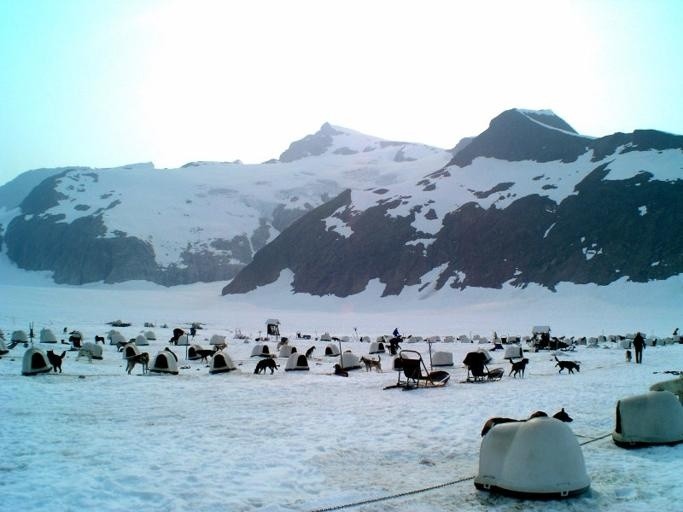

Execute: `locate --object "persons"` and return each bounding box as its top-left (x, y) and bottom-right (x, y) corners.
top-left (389, 337), bottom-right (403, 354)
top-left (393, 328), bottom-right (399, 336)
top-left (633, 332), bottom-right (646, 363)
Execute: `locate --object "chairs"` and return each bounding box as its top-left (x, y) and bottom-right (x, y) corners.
top-left (465, 351), bottom-right (504, 381)
top-left (397, 350), bottom-right (449, 387)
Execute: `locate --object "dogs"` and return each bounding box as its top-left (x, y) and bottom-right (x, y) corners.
top-left (254, 359), bottom-right (278, 375)
top-left (305, 345), bottom-right (315, 358)
top-left (122, 352), bottom-right (149, 375)
top-left (555, 356), bottom-right (580, 374)
top-left (194, 350), bottom-right (216, 364)
top-left (359, 355), bottom-right (383, 373)
top-left (47, 349), bottom-right (66, 373)
top-left (384, 343), bottom-right (401, 356)
top-left (333, 364), bottom-right (348, 377)
top-left (116, 338), bottom-right (136, 352)
top-left (481, 407), bottom-right (573, 437)
top-left (509, 357), bottom-right (529, 378)
top-left (94, 334), bottom-right (105, 344)
